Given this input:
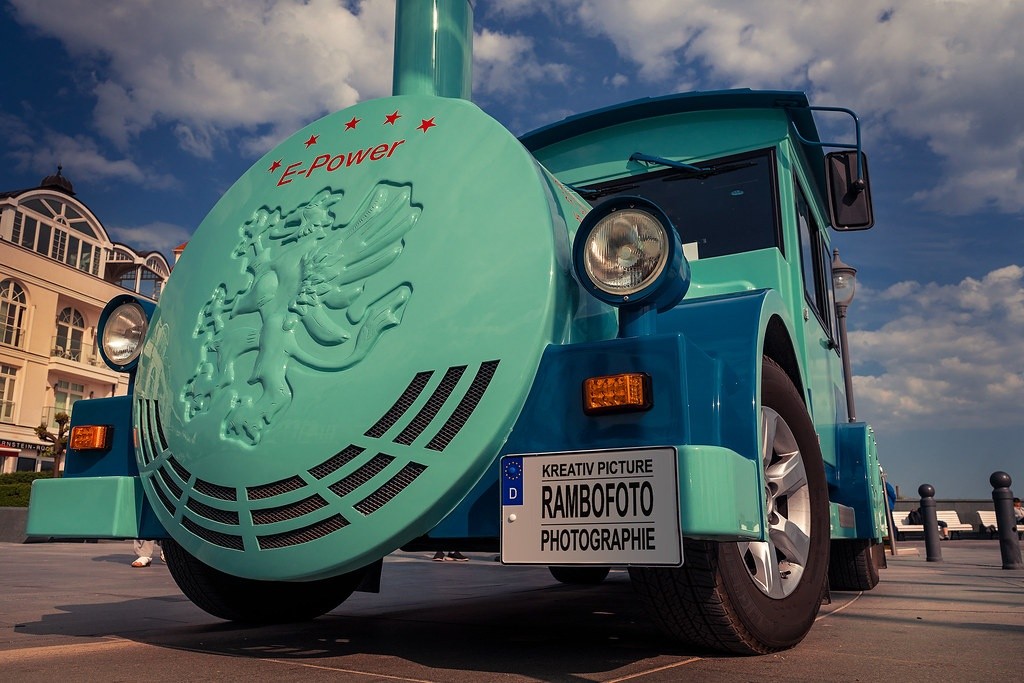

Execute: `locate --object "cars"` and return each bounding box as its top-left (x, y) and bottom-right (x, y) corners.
top-left (24, 2), bottom-right (888, 655)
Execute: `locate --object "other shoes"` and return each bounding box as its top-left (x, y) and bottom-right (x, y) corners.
top-left (160, 552), bottom-right (167, 565)
top-left (945, 535), bottom-right (950, 540)
top-left (132, 557), bottom-right (152, 567)
top-left (939, 535), bottom-right (944, 540)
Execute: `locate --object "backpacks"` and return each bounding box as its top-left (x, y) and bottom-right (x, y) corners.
top-left (909, 509), bottom-right (922, 525)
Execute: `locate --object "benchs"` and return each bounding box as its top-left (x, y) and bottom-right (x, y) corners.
top-left (976, 510), bottom-right (1024, 539)
top-left (892, 511), bottom-right (973, 540)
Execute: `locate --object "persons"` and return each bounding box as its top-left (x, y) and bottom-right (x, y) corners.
top-left (1013, 498), bottom-right (1024, 540)
top-left (431, 552), bottom-right (469, 564)
top-left (908, 508), bottom-right (951, 542)
top-left (882, 481), bottom-right (898, 542)
top-left (131, 539), bottom-right (166, 568)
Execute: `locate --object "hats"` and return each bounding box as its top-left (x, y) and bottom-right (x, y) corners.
top-left (1013, 498), bottom-right (1021, 503)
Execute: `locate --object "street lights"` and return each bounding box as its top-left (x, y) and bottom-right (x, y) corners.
top-left (832, 248), bottom-right (857, 422)
